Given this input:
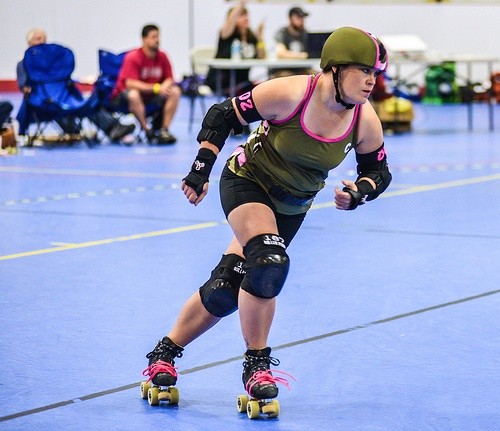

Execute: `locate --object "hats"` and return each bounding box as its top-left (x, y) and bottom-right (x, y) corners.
top-left (289, 7), bottom-right (308, 17)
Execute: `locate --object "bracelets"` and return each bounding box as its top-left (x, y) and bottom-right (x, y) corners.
top-left (152, 84), bottom-right (160, 94)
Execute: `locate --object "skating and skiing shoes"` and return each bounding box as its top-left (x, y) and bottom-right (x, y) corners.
top-left (139, 336), bottom-right (186, 406)
top-left (236, 346), bottom-right (297, 419)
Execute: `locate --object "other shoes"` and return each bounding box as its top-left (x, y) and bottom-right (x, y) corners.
top-left (147, 129), bottom-right (159, 146)
top-left (158, 127), bottom-right (175, 145)
top-left (109, 124), bottom-right (135, 142)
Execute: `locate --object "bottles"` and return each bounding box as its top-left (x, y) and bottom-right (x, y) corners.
top-left (231, 38), bottom-right (241, 61)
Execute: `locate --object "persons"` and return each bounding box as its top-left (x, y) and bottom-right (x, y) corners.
top-left (203, 0), bottom-right (266, 137)
top-left (269, 7), bottom-right (321, 79)
top-left (140, 27), bottom-right (392, 418)
top-left (16, 27), bottom-right (135, 145)
top-left (110, 25), bottom-right (182, 144)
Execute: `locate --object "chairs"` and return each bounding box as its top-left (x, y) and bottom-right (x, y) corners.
top-left (98, 50), bottom-right (160, 146)
top-left (190, 47), bottom-right (213, 138)
top-left (23, 44), bottom-right (100, 147)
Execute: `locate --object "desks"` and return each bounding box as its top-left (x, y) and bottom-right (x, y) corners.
top-left (200, 58), bottom-right (323, 102)
top-left (388, 55), bottom-right (500, 132)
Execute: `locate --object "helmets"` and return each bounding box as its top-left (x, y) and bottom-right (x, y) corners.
top-left (320, 27), bottom-right (389, 72)
